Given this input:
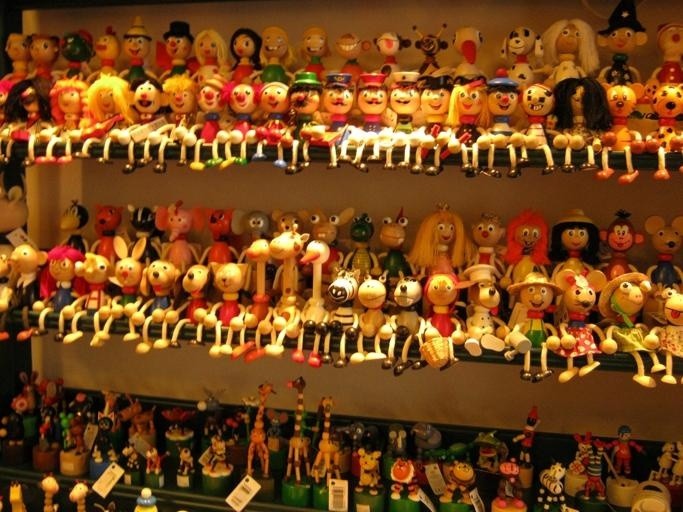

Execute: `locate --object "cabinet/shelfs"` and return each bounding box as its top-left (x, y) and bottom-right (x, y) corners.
top-left (0, 106), bottom-right (683, 511)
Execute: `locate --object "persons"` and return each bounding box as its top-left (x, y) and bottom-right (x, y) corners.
top-left (0, 0), bottom-right (683, 512)
